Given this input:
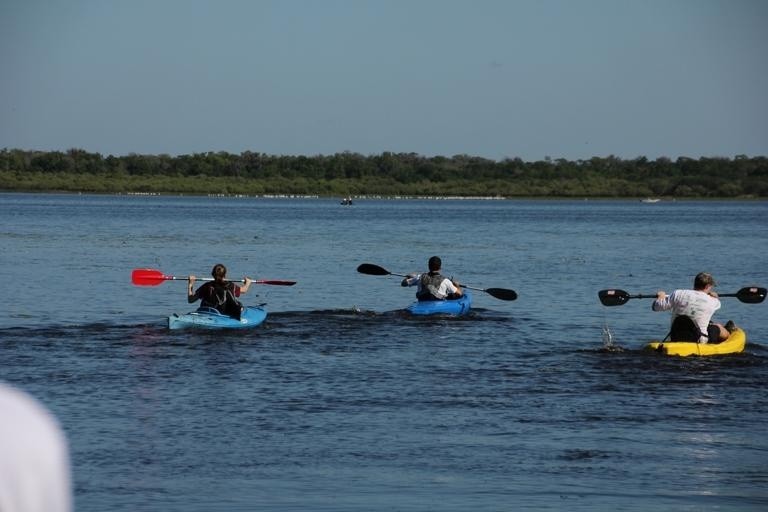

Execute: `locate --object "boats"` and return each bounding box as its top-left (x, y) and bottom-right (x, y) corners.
top-left (341, 199), bottom-right (356, 206)
top-left (640, 199), bottom-right (661, 203)
top-left (168, 302), bottom-right (269, 330)
top-left (642, 325), bottom-right (746, 357)
top-left (404, 289), bottom-right (472, 316)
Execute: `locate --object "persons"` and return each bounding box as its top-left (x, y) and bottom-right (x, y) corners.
top-left (400, 256), bottom-right (463, 300)
top-left (186, 263), bottom-right (252, 321)
top-left (650, 273), bottom-right (737, 343)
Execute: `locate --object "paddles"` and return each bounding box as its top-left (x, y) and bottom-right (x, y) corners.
top-left (598, 287), bottom-right (768, 306)
top-left (357, 264), bottom-right (517, 300)
top-left (132, 269), bottom-right (296, 286)
